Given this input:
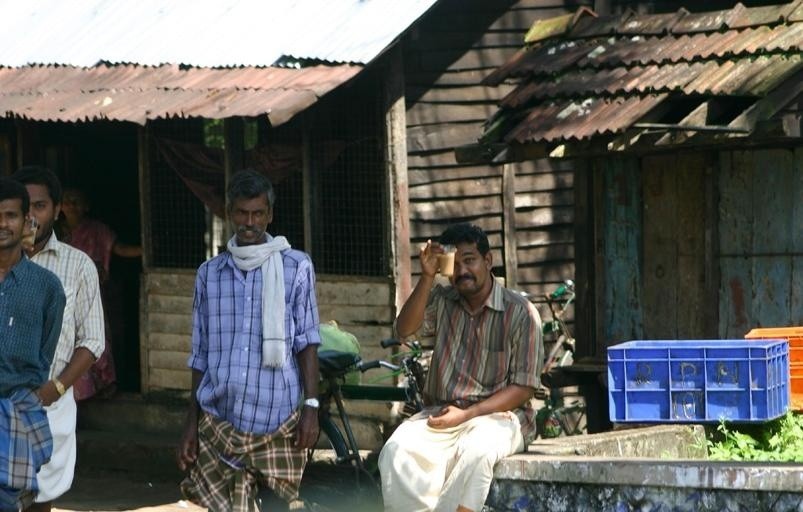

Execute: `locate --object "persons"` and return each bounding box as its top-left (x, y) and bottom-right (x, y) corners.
top-left (175, 169), bottom-right (320, 512)
top-left (1, 178), bottom-right (68, 512)
top-left (11, 167), bottom-right (107, 511)
top-left (377, 223), bottom-right (542, 512)
top-left (55, 187), bottom-right (143, 400)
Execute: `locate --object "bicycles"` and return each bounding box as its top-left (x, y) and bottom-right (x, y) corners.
top-left (523, 280), bottom-right (588, 435)
top-left (297, 321), bottom-right (434, 480)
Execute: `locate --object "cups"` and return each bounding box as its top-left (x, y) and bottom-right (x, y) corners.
top-left (435, 245), bottom-right (456, 276)
top-left (22, 218), bottom-right (37, 250)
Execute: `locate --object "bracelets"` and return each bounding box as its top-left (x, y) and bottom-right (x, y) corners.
top-left (52, 379), bottom-right (66, 395)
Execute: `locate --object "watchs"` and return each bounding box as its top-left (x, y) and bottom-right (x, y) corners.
top-left (304, 397), bottom-right (319, 409)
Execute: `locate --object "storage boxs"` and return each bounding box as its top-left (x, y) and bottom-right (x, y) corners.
top-left (606, 326), bottom-right (802, 426)
